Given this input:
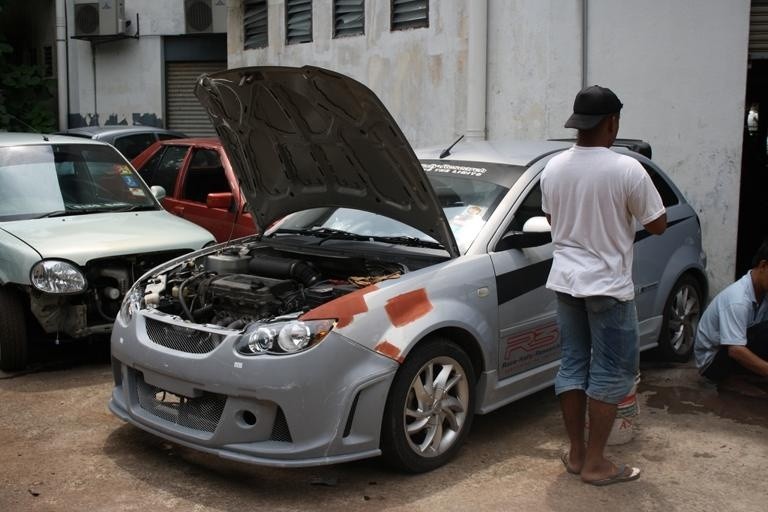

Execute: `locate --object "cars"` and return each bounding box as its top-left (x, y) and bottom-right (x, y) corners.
top-left (0, 130), bottom-right (219, 372)
top-left (109, 64), bottom-right (711, 475)
top-left (47, 124), bottom-right (191, 185)
top-left (99, 137), bottom-right (258, 244)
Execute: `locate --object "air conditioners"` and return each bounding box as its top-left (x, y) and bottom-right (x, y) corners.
top-left (73, 1), bottom-right (126, 35)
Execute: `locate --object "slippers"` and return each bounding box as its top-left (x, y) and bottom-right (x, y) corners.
top-left (590, 465), bottom-right (641, 485)
top-left (561, 446), bottom-right (579, 474)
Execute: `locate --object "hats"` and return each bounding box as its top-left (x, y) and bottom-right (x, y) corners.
top-left (563, 85), bottom-right (622, 130)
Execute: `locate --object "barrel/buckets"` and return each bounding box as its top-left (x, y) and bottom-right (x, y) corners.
top-left (584, 370), bottom-right (641, 445)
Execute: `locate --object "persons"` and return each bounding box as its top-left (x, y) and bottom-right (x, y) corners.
top-left (695, 246), bottom-right (768, 382)
top-left (541, 85), bottom-right (666, 487)
